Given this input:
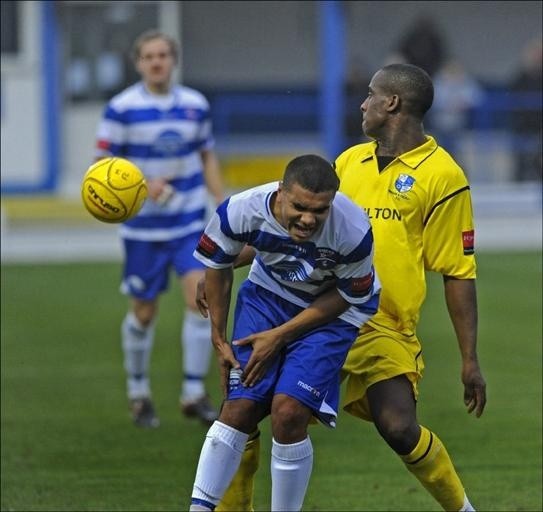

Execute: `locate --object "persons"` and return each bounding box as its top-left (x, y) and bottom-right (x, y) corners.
top-left (95, 29), bottom-right (225, 427)
top-left (386, 19), bottom-right (543, 176)
top-left (196, 63), bottom-right (487, 512)
top-left (187, 154), bottom-right (382, 512)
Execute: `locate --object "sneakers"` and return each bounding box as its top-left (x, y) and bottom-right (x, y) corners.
top-left (178, 394), bottom-right (219, 426)
top-left (128, 397), bottom-right (159, 429)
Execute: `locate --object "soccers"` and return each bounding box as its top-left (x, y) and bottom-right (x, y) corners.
top-left (83, 157), bottom-right (148, 223)
